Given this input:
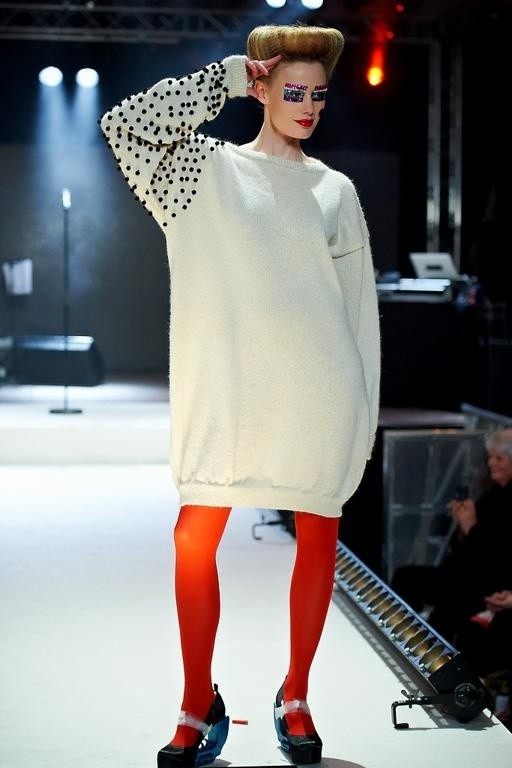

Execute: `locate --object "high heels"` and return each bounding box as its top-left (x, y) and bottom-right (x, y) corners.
top-left (272, 675), bottom-right (323, 765)
top-left (156, 684), bottom-right (231, 768)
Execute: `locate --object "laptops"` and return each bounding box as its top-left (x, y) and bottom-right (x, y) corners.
top-left (409, 253), bottom-right (469, 281)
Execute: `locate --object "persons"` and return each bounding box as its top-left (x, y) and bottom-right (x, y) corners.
top-left (384, 425), bottom-right (511, 647)
top-left (457, 590), bottom-right (511, 700)
top-left (96, 21), bottom-right (385, 768)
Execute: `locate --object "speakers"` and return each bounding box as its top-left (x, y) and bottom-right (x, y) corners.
top-left (13, 334), bottom-right (104, 387)
